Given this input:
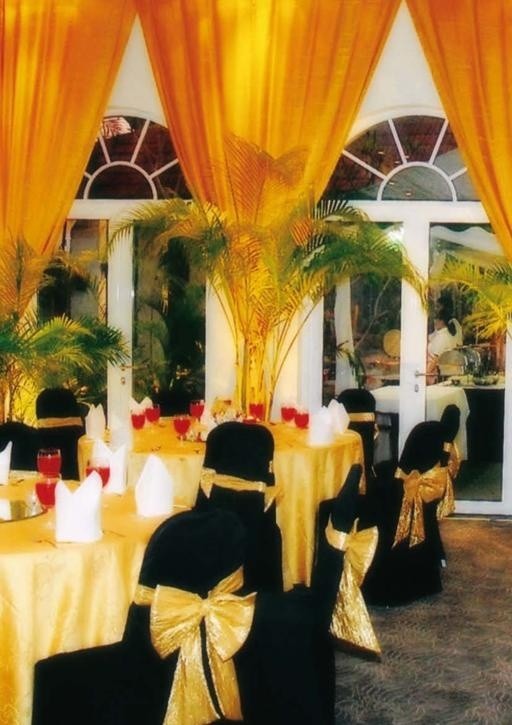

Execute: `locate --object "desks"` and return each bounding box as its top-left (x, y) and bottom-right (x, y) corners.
top-left (427, 371), bottom-right (505, 463)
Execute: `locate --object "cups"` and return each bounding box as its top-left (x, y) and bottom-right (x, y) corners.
top-left (85, 457), bottom-right (111, 490)
top-left (35, 447), bottom-right (64, 476)
top-left (131, 401), bottom-right (163, 431)
top-left (243, 402), bottom-right (309, 430)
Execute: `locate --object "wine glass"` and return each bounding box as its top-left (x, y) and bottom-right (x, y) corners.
top-left (36, 473), bottom-right (62, 530)
top-left (173, 399), bottom-right (205, 450)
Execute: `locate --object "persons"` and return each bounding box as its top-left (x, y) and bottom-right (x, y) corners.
top-left (427, 308), bottom-right (456, 385)
top-left (436, 296), bottom-right (463, 346)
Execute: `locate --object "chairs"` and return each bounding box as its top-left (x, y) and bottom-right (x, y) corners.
top-left (34, 386), bottom-right (460, 605)
top-left (1, 422), bottom-right (363, 725)
